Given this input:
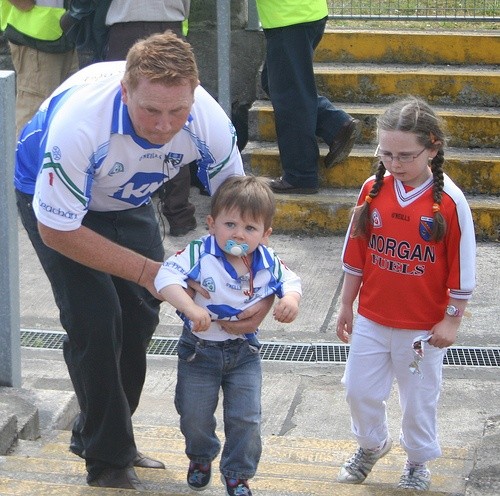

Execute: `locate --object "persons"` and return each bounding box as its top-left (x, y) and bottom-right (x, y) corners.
top-left (106, 0), bottom-right (190, 61)
top-left (255, 0), bottom-right (363, 195)
top-left (14, 29), bottom-right (275, 491)
top-left (0, 0), bottom-right (81, 144)
top-left (336, 97), bottom-right (476, 492)
top-left (154, 174), bottom-right (302, 495)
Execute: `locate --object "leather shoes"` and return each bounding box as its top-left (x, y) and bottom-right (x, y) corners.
top-left (96, 466), bottom-right (145, 490)
top-left (265, 176), bottom-right (318, 194)
top-left (72, 451), bottom-right (165, 468)
top-left (322, 116), bottom-right (362, 168)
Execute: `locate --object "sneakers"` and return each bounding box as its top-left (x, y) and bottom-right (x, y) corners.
top-left (399, 466), bottom-right (431, 490)
top-left (220, 473), bottom-right (252, 496)
top-left (168, 215), bottom-right (195, 236)
top-left (337, 434), bottom-right (391, 483)
top-left (186, 460), bottom-right (212, 491)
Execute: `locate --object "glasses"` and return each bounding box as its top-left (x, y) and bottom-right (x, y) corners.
top-left (373, 144), bottom-right (428, 163)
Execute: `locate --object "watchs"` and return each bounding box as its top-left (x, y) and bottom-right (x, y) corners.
top-left (445, 305), bottom-right (465, 319)
